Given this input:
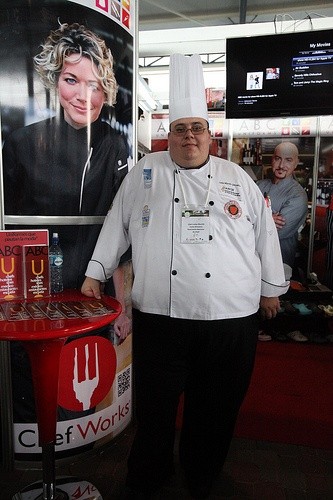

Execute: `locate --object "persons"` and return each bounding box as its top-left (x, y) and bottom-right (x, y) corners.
top-left (80, 98), bottom-right (290, 500)
top-left (2, 23), bottom-right (134, 217)
top-left (254, 143), bottom-right (309, 280)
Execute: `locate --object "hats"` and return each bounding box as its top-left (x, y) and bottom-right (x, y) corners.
top-left (169, 53), bottom-right (210, 126)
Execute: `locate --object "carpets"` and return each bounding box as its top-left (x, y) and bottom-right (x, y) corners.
top-left (169, 330), bottom-right (333, 453)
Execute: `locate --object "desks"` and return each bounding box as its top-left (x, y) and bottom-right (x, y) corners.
top-left (0, 290), bottom-right (123, 500)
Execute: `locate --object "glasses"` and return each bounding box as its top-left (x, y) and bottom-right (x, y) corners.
top-left (170, 126), bottom-right (209, 136)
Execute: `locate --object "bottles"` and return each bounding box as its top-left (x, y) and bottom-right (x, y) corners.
top-left (243, 143), bottom-right (256, 165)
top-left (304, 180), bottom-right (331, 204)
top-left (49, 233), bottom-right (63, 294)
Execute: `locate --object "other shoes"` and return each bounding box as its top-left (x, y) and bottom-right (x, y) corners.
top-left (185, 477), bottom-right (210, 500)
top-left (257, 329), bottom-right (272, 341)
top-left (287, 330), bottom-right (308, 343)
top-left (278, 297), bottom-right (333, 320)
top-left (115, 483), bottom-right (148, 499)
